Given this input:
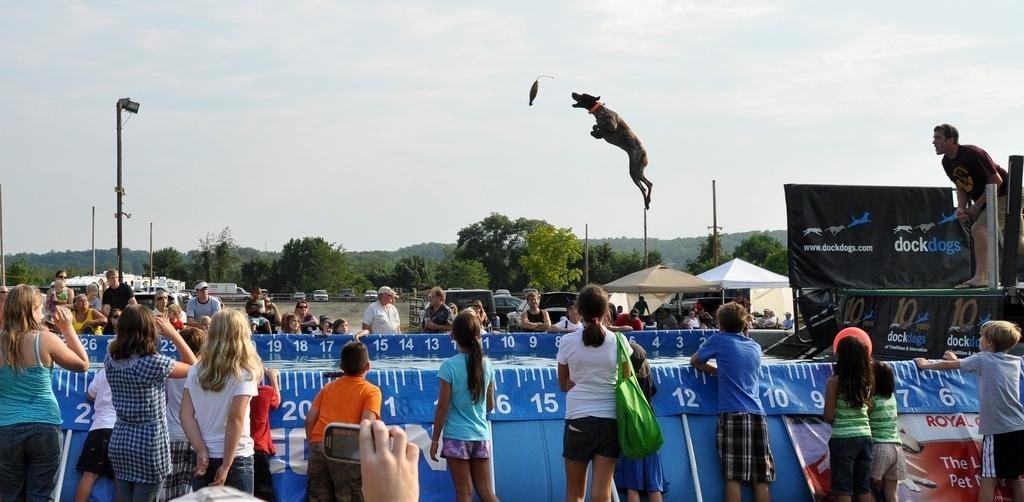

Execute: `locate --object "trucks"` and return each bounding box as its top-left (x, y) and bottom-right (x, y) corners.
top-left (202, 281), bottom-right (252, 302)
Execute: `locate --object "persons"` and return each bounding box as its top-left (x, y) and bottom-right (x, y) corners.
top-left (615, 343), bottom-right (669, 502)
top-left (306, 340), bottom-right (383, 502)
top-left (75, 305), bottom-right (280, 501)
top-left (932, 125), bottom-right (1024, 289)
top-left (353, 286), bottom-right (401, 341)
top-left (868, 361), bottom-right (907, 502)
top-left (824, 335), bottom-right (875, 502)
top-left (913, 320), bottom-right (1024, 502)
top-left (0, 283), bottom-right (89, 502)
top-left (44, 271), bottom-right (349, 335)
top-left (359, 419), bottom-right (420, 502)
top-left (690, 300), bottom-right (776, 502)
top-left (430, 312), bottom-right (500, 502)
top-left (557, 283), bottom-right (634, 502)
top-left (424, 289), bottom-right (794, 341)
top-left (1, 287), bottom-right (8, 301)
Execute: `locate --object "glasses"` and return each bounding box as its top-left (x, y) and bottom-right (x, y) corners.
top-left (60, 276), bottom-right (68, 278)
top-left (299, 306), bottom-right (307, 308)
top-left (112, 314), bottom-right (119, 317)
top-left (158, 296), bottom-right (168, 299)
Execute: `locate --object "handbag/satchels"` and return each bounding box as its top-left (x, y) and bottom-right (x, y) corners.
top-left (615, 376), bottom-right (663, 460)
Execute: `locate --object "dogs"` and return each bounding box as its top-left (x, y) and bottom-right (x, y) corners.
top-left (571, 92), bottom-right (654, 210)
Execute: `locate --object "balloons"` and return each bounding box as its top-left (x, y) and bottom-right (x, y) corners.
top-left (833, 326), bottom-right (872, 357)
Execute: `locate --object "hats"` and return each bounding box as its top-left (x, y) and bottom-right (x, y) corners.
top-left (194, 281), bottom-right (209, 291)
top-left (630, 343), bottom-right (647, 371)
top-left (377, 286), bottom-right (396, 296)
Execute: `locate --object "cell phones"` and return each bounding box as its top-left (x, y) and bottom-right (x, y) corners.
top-left (321, 422), bottom-right (395, 464)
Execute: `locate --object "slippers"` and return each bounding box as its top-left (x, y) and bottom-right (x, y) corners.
top-left (954, 281), bottom-right (988, 289)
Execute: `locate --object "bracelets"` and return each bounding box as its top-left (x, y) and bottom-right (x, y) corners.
top-left (432, 441), bottom-right (438, 444)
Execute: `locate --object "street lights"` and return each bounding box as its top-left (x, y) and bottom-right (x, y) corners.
top-left (113, 93), bottom-right (140, 284)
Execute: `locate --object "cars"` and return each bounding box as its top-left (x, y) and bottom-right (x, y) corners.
top-left (490, 292), bottom-right (581, 331)
top-left (313, 288), bottom-right (328, 302)
top-left (339, 288), bottom-right (355, 301)
top-left (363, 289), bottom-right (379, 302)
top-left (292, 290), bottom-right (306, 300)
top-left (133, 286), bottom-right (194, 313)
top-left (447, 286), bottom-right (540, 293)
top-left (440, 288), bottom-right (500, 332)
